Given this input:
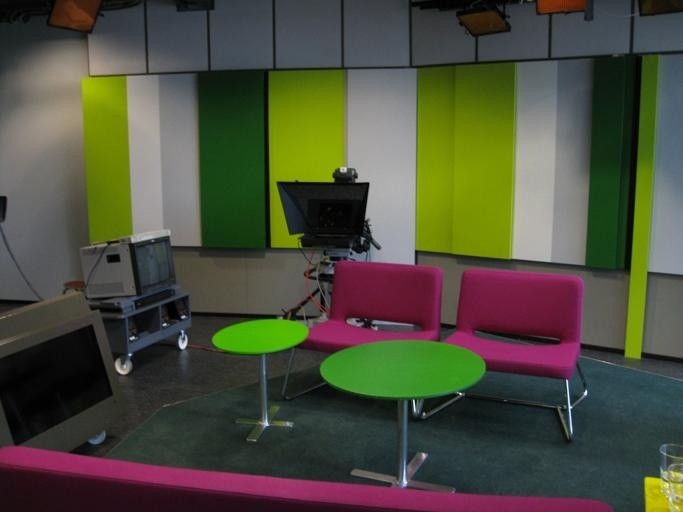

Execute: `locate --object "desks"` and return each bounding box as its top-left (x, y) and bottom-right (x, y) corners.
top-left (210, 317), bottom-right (490, 497)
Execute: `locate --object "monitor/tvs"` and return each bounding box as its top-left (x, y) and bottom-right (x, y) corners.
top-left (79, 235), bottom-right (177, 300)
top-left (0, 288), bottom-right (127, 456)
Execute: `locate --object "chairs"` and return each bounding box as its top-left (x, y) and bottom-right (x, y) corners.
top-left (417, 269), bottom-right (587, 442)
top-left (281, 261), bottom-right (443, 418)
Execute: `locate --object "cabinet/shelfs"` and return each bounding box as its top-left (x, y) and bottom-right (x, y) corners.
top-left (88, 290), bottom-right (193, 375)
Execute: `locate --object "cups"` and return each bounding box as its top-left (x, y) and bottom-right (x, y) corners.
top-left (666, 462), bottom-right (683, 512)
top-left (659, 443), bottom-right (683, 500)
